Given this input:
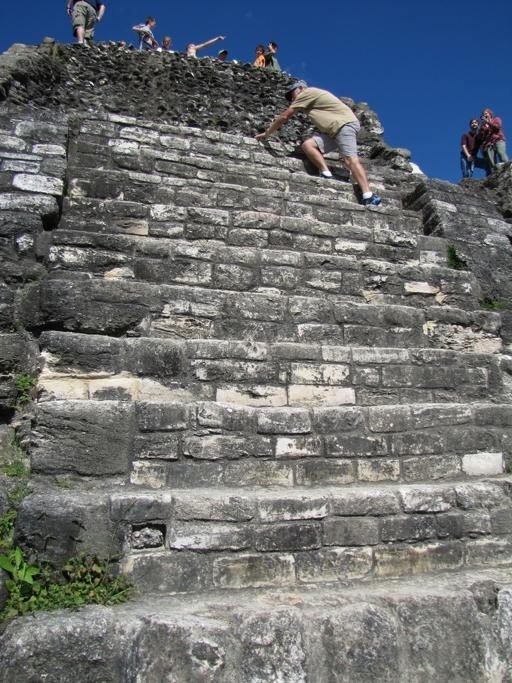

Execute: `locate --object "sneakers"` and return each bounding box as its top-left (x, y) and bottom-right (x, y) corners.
top-left (362, 191), bottom-right (381, 206)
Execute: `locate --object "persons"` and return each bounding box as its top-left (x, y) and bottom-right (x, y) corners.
top-left (218, 49), bottom-right (228, 60)
top-left (255, 79), bottom-right (381, 204)
top-left (459, 118), bottom-right (491, 178)
top-left (66, 0), bottom-right (106, 42)
top-left (132, 14), bottom-right (159, 50)
top-left (252, 44), bottom-right (266, 67)
top-left (186, 34), bottom-right (224, 57)
top-left (264, 40), bottom-right (281, 70)
top-left (479, 107), bottom-right (508, 173)
top-left (161, 35), bottom-right (174, 52)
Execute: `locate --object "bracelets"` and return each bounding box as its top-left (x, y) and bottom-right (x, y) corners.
top-left (67, 7), bottom-right (71, 9)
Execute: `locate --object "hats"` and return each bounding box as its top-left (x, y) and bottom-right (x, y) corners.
top-left (218, 49), bottom-right (228, 55)
top-left (278, 78), bottom-right (308, 94)
